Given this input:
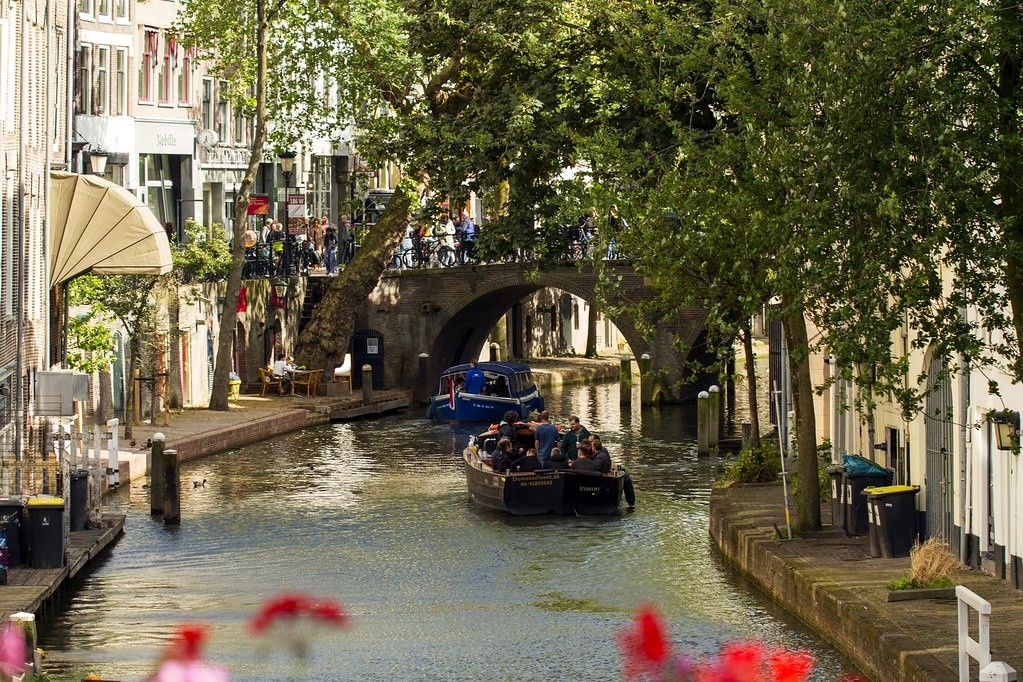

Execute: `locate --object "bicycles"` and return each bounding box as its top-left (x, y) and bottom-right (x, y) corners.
top-left (385, 230), bottom-right (636, 271)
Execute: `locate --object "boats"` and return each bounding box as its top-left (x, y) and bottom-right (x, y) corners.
top-left (462, 420), bottom-right (626, 518)
top-left (425, 360), bottom-right (545, 424)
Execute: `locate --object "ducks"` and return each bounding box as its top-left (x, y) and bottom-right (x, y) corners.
top-left (129, 438), bottom-right (138, 448)
top-left (193, 478), bottom-right (208, 486)
top-left (147, 438), bottom-right (153, 450)
top-left (308, 463), bottom-right (314, 470)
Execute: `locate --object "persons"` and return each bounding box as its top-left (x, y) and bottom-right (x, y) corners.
top-left (462, 359), bottom-right (486, 395)
top-left (243, 214), bottom-right (355, 275)
top-left (454, 377), bottom-right (465, 393)
top-left (489, 376), bottom-right (509, 397)
top-left (397, 211), bottom-right (475, 268)
top-left (273, 354), bottom-right (294, 397)
top-left (490, 411), bottom-right (611, 473)
top-left (165, 222), bottom-right (175, 243)
top-left (311, 276), bottom-right (331, 318)
top-left (566, 203), bottom-right (631, 260)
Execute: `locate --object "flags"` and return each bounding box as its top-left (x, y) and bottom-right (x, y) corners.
top-left (448, 375), bottom-right (455, 410)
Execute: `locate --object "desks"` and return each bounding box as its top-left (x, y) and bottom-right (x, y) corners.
top-left (267, 367), bottom-right (314, 392)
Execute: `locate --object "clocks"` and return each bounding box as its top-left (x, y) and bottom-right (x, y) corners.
top-left (197, 128), bottom-right (216, 146)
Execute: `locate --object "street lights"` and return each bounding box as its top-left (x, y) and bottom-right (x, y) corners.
top-left (278, 150), bottom-right (296, 276)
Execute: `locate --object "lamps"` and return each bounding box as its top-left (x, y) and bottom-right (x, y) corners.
top-left (992, 411), bottom-right (1019, 450)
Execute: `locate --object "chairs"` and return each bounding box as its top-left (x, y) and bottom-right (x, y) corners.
top-left (292, 369), bottom-right (325, 398)
top-left (258, 367), bottom-right (283, 398)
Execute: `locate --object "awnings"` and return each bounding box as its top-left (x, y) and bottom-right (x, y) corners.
top-left (51, 170), bottom-right (173, 289)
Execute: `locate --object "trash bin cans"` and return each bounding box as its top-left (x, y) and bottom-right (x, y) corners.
top-left (842, 472), bottom-right (893, 537)
top-left (835, 468), bottom-right (848, 531)
top-left (26, 497), bottom-right (68, 570)
top-left (58, 470), bottom-right (92, 532)
top-left (867, 485), bottom-right (921, 558)
top-left (827, 469), bottom-right (840, 528)
top-left (861, 486), bottom-right (901, 558)
top-left (0, 500), bottom-right (27, 569)
